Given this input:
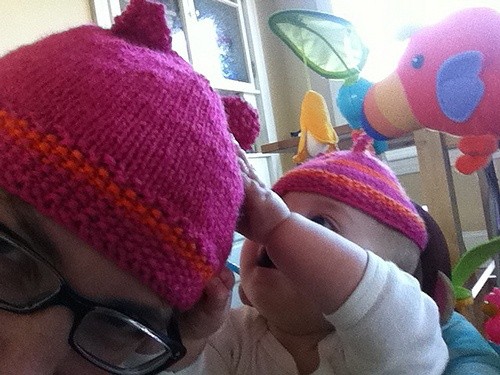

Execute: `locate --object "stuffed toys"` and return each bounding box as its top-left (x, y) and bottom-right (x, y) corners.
top-left (357, 8), bottom-right (500, 176)
top-left (292, 89), bottom-right (340, 164)
top-left (336, 75), bottom-right (392, 155)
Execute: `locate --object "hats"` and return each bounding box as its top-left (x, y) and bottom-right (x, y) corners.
top-left (269, 131), bottom-right (428, 251)
top-left (0, 0), bottom-right (260, 310)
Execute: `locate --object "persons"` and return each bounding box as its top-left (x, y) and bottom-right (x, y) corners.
top-left (0, 0), bottom-right (260, 375)
top-left (200, 126), bottom-right (455, 375)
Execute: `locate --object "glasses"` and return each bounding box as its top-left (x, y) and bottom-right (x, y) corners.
top-left (0, 224), bottom-right (186, 375)
